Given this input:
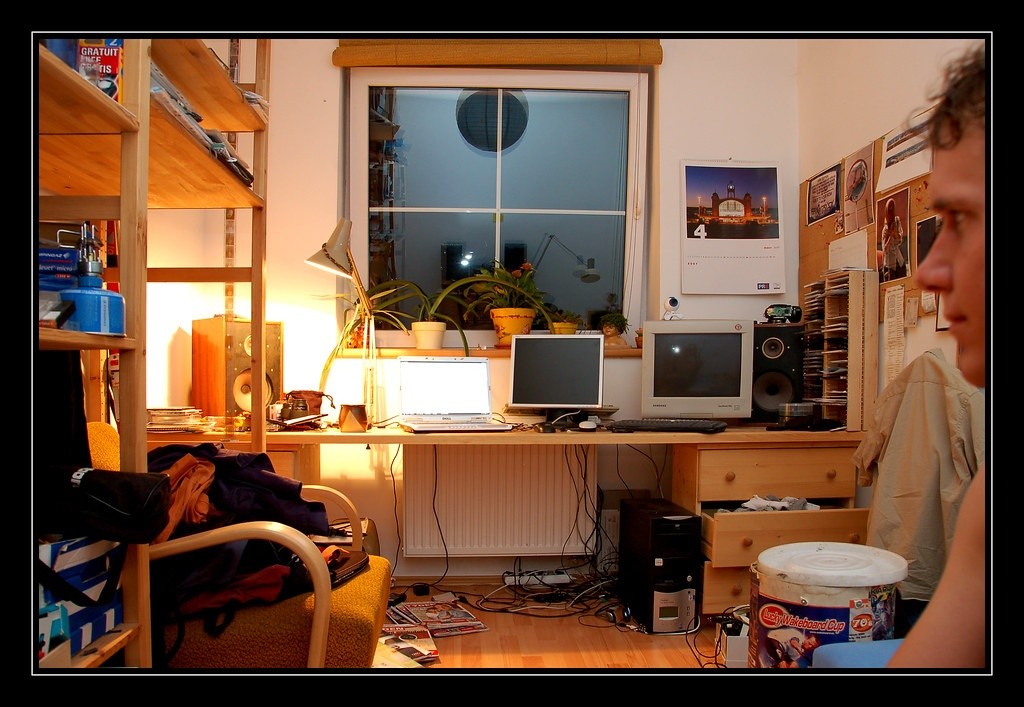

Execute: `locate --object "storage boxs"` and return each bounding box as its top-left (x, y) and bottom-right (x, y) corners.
top-left (39, 247), bottom-right (80, 329)
top-left (38, 534), bottom-right (128, 661)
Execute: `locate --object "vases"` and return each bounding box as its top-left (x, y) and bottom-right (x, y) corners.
top-left (363, 315), bottom-right (387, 433)
top-left (489, 307), bottom-right (539, 350)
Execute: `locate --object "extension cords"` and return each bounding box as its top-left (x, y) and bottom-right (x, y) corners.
top-left (504, 571), bottom-right (570, 585)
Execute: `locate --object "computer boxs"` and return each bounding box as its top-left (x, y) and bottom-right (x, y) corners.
top-left (618, 497), bottom-right (702, 634)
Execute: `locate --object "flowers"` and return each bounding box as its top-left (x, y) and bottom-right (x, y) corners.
top-left (464, 259), bottom-right (537, 303)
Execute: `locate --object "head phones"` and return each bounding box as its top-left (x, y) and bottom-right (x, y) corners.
top-left (595, 602), bottom-right (631, 623)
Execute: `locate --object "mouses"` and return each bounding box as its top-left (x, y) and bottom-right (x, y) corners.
top-left (579, 421), bottom-right (597, 432)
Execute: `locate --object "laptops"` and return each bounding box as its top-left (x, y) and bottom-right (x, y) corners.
top-left (398, 356), bottom-right (512, 433)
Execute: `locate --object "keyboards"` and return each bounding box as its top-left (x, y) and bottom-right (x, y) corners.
top-left (606, 418), bottom-right (727, 434)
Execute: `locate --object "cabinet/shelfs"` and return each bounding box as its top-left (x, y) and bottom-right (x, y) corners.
top-left (39, 33), bottom-right (277, 668)
top-left (798, 270), bottom-right (880, 433)
top-left (369, 85), bottom-right (401, 329)
top-left (146, 418), bottom-right (874, 625)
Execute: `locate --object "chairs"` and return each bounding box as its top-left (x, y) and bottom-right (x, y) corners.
top-left (86, 421), bottom-right (394, 669)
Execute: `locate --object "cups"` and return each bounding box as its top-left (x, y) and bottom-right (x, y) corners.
top-left (339, 404), bottom-right (368, 433)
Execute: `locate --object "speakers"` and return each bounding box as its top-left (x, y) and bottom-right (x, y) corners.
top-left (191, 317), bottom-right (283, 431)
top-left (752, 327), bottom-right (804, 423)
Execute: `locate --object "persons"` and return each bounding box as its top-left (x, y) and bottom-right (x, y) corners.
top-left (881, 199), bottom-right (907, 281)
top-left (871, 586), bottom-right (901, 640)
top-left (775, 634), bottom-right (820, 668)
top-left (602, 313), bottom-right (630, 345)
top-left (887, 42), bottom-right (985, 667)
top-left (441, 603), bottom-right (474, 618)
top-left (425, 607), bottom-right (440, 620)
top-left (748, 637), bottom-right (765, 669)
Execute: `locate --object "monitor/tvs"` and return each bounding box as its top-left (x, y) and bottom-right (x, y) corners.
top-left (641, 319), bottom-right (754, 419)
top-left (510, 334), bottom-right (605, 430)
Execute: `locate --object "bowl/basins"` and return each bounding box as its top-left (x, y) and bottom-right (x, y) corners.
top-left (777, 403), bottom-right (815, 425)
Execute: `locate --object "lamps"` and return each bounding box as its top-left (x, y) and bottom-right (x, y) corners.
top-left (456, 89), bottom-right (527, 152)
top-left (532, 233), bottom-right (601, 283)
top-left (303, 214), bottom-right (376, 432)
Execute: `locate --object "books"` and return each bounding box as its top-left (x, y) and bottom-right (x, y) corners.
top-left (377, 601), bottom-right (489, 662)
top-left (146, 405), bottom-right (217, 433)
top-left (38, 247), bottom-right (80, 290)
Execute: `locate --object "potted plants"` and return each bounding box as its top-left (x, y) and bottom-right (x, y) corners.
top-left (396, 273), bottom-right (468, 349)
top-left (548, 307), bottom-right (591, 335)
top-left (600, 314), bottom-right (634, 349)
top-left (635, 327), bottom-right (643, 349)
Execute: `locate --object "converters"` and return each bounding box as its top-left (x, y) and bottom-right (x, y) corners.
top-left (388, 593), bottom-right (405, 607)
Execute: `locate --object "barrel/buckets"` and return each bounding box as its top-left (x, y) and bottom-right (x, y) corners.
top-left (746, 542), bottom-right (909, 668)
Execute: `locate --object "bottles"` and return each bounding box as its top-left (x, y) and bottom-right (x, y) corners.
top-left (280, 399), bottom-right (309, 422)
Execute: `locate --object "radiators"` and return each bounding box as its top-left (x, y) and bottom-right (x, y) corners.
top-left (401, 444), bottom-right (597, 558)
